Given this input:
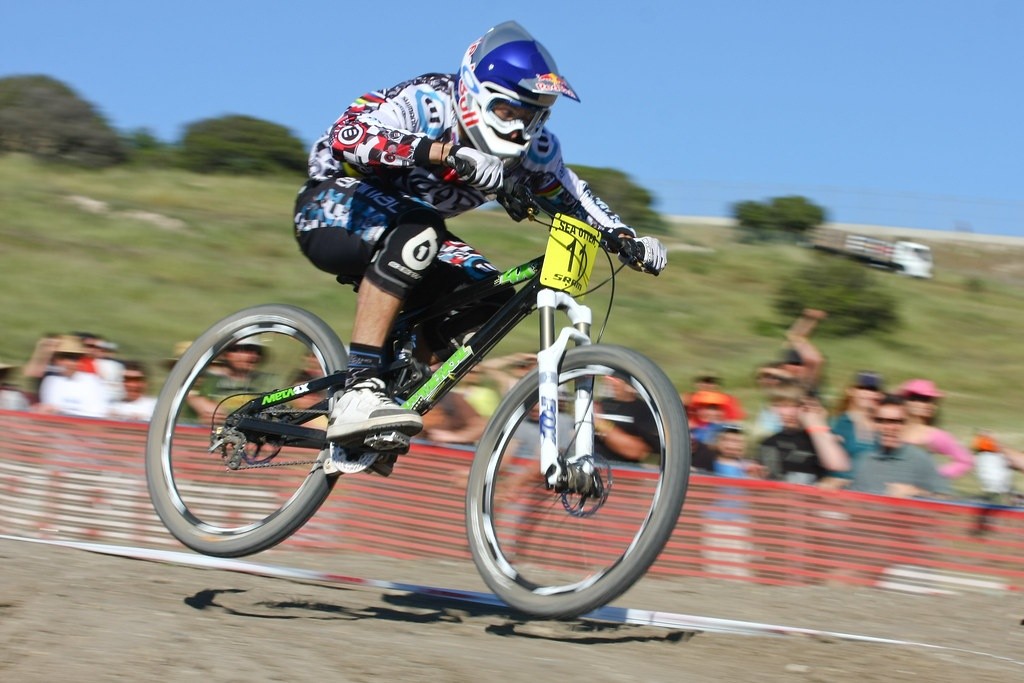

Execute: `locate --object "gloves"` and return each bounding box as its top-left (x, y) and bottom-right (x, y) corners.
top-left (618, 236), bottom-right (668, 273)
top-left (447, 144), bottom-right (504, 194)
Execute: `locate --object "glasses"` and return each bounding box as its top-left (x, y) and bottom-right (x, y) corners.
top-left (54, 351), bottom-right (81, 361)
top-left (481, 93), bottom-right (551, 140)
top-left (871, 416), bottom-right (901, 423)
top-left (120, 374), bottom-right (144, 379)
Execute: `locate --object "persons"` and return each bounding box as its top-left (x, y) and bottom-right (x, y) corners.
top-left (292, 20), bottom-right (667, 442)
top-left (1, 303), bottom-right (1024, 607)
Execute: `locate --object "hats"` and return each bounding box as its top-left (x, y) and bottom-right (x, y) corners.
top-left (900, 379), bottom-right (940, 396)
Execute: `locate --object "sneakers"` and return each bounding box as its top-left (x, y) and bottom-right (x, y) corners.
top-left (326, 378), bottom-right (423, 447)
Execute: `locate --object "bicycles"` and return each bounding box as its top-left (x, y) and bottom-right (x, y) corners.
top-left (136, 158), bottom-right (697, 620)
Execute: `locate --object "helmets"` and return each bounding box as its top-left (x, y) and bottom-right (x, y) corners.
top-left (217, 324), bottom-right (270, 365)
top-left (454, 21), bottom-right (580, 169)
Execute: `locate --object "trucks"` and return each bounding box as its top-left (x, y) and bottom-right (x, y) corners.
top-left (843, 230), bottom-right (936, 280)
